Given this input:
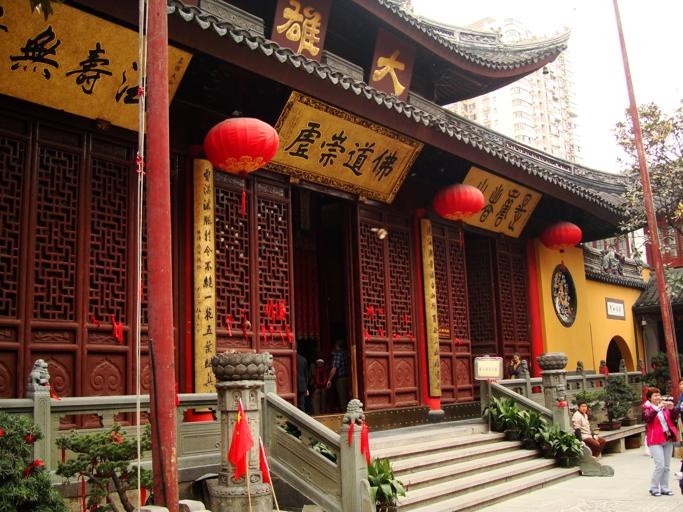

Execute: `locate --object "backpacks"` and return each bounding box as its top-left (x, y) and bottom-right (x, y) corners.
top-left (311, 361), bottom-right (328, 389)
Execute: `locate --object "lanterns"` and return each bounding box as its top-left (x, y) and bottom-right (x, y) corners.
top-left (201, 110), bottom-right (280, 218)
top-left (536, 219), bottom-right (583, 272)
top-left (431, 179), bottom-right (485, 252)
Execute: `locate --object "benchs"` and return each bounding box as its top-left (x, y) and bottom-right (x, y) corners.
top-left (589, 425), bottom-right (647, 455)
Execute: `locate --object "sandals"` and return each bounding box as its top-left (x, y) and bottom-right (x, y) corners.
top-left (650, 490), bottom-right (674, 496)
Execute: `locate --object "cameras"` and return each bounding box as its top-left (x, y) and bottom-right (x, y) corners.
top-left (664, 403), bottom-right (669, 407)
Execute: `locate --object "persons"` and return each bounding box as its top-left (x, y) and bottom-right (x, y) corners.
top-left (641, 387), bottom-right (678, 497)
top-left (294, 339), bottom-right (311, 413)
top-left (570, 398), bottom-right (607, 457)
top-left (505, 351), bottom-right (523, 379)
top-left (324, 339), bottom-right (353, 413)
top-left (673, 379), bottom-right (683, 430)
top-left (307, 358), bottom-right (330, 414)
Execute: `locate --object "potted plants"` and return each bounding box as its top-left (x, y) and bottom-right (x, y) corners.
top-left (487, 395), bottom-right (580, 468)
top-left (597, 375), bottom-right (642, 430)
top-left (369, 460), bottom-right (409, 512)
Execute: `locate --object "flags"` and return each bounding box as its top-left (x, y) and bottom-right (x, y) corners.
top-left (227, 400), bottom-right (254, 482)
top-left (257, 440), bottom-right (271, 484)
